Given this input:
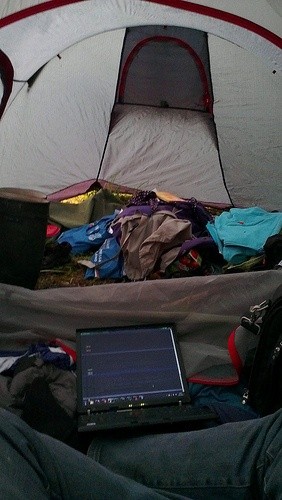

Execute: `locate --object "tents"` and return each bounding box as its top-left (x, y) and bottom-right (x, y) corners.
top-left (0, 0), bottom-right (281, 379)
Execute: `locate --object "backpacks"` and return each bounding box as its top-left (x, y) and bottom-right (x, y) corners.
top-left (240, 284), bottom-right (282, 416)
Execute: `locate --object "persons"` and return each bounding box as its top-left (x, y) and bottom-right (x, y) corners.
top-left (0, 405), bottom-right (282, 500)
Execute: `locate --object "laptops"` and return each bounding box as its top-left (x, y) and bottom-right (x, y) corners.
top-left (72, 321), bottom-right (219, 432)
